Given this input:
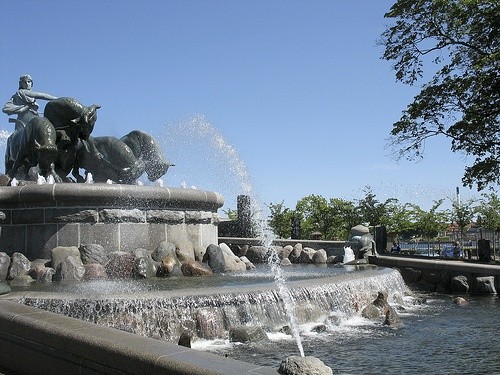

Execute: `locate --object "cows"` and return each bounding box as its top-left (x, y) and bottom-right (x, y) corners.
top-left (4, 97), bottom-right (176, 183)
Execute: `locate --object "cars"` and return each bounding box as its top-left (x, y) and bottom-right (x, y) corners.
top-left (391, 242), bottom-right (416, 255)
top-left (441, 245), bottom-right (464, 259)
top-left (414, 247), bottom-right (439, 258)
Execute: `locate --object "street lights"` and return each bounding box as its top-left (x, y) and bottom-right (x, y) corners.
top-left (456, 186), bottom-right (460, 209)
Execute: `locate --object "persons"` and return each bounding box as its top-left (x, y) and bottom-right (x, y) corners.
top-left (3, 75), bottom-right (60, 128)
top-left (391, 234), bottom-right (400, 253)
